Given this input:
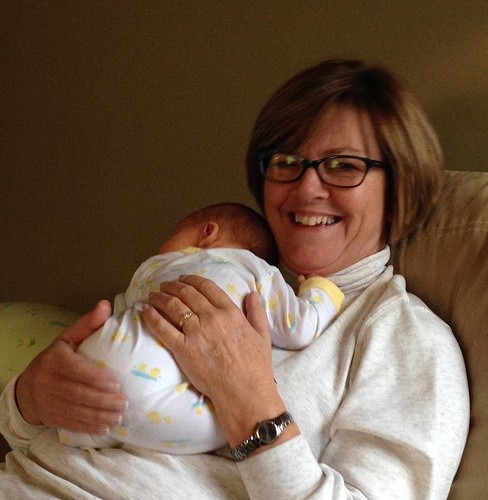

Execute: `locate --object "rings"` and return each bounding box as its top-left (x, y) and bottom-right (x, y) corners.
top-left (178, 311), bottom-right (194, 329)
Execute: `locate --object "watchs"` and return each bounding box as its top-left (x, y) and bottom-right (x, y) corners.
top-left (231, 411), bottom-right (294, 463)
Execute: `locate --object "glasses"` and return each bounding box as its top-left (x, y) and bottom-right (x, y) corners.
top-left (257, 150), bottom-right (389, 188)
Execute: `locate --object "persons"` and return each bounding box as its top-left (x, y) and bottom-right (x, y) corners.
top-left (57, 202), bottom-right (345, 458)
top-left (0, 55), bottom-right (470, 500)
